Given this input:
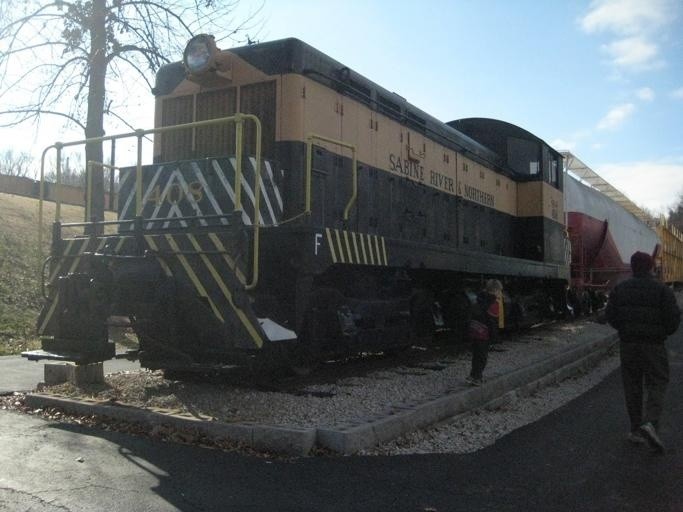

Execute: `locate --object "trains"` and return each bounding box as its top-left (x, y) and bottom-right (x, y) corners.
top-left (18, 32), bottom-right (683, 380)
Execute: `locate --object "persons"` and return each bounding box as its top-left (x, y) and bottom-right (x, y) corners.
top-left (604, 251), bottom-right (680, 450)
top-left (464, 278), bottom-right (503, 386)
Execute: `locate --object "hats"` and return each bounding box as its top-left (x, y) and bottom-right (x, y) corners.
top-left (631, 251), bottom-right (653, 272)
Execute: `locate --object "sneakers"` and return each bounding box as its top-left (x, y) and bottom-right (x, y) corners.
top-left (628, 422), bottom-right (666, 453)
top-left (465, 377), bottom-right (482, 385)
